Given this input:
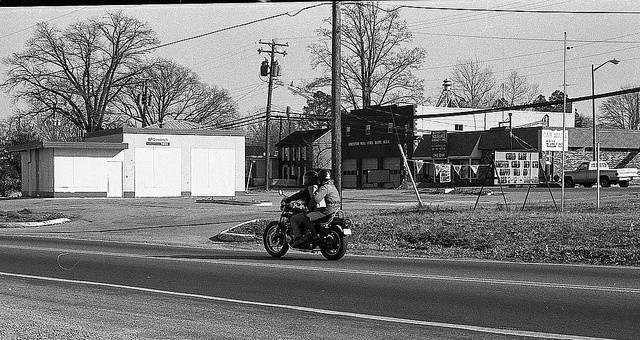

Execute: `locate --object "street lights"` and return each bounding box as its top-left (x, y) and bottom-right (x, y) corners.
top-left (592, 58), bottom-right (620, 209)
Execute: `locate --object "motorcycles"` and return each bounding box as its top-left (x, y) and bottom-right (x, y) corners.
top-left (263, 189), bottom-right (352, 261)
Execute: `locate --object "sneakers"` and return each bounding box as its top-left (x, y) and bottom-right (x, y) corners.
top-left (310, 236), bottom-right (320, 244)
top-left (290, 236), bottom-right (304, 249)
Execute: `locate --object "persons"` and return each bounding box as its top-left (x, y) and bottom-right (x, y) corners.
top-left (284, 170), bottom-right (319, 246)
top-left (301, 169), bottom-right (341, 240)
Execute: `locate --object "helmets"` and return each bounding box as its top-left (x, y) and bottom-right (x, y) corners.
top-left (319, 169), bottom-right (331, 181)
top-left (303, 170), bottom-right (320, 186)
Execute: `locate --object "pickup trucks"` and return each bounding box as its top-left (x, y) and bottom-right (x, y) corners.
top-left (561, 161), bottom-right (638, 188)
